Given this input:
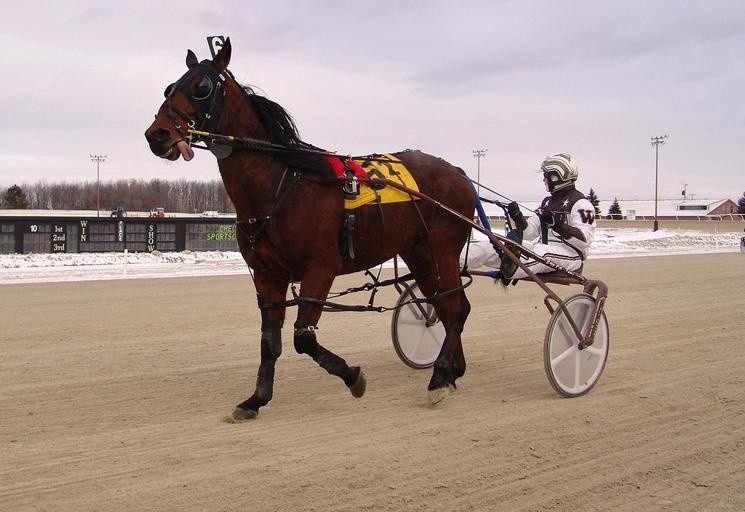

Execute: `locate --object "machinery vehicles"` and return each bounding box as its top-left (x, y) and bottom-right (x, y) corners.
top-left (149, 206), bottom-right (166, 218)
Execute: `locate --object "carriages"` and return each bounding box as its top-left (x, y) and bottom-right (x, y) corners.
top-left (147, 33), bottom-right (616, 422)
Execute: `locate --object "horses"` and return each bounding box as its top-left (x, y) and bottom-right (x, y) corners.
top-left (143, 36), bottom-right (478, 421)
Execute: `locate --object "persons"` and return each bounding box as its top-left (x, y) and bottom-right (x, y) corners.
top-left (459, 152), bottom-right (596, 279)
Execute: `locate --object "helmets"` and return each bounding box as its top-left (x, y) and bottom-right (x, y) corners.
top-left (540, 152), bottom-right (579, 191)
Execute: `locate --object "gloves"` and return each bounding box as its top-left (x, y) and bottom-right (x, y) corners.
top-left (508, 201), bottom-right (522, 223)
top-left (543, 211), bottom-right (561, 231)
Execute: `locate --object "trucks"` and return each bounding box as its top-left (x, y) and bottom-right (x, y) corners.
top-left (200, 211), bottom-right (218, 218)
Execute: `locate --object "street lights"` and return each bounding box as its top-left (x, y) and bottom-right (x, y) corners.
top-left (88, 152), bottom-right (108, 220)
top-left (648, 134), bottom-right (667, 232)
top-left (470, 147), bottom-right (488, 227)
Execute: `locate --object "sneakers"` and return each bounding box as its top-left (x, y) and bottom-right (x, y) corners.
top-left (501, 232), bottom-right (521, 276)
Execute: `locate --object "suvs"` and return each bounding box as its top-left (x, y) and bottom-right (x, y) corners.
top-left (109, 205), bottom-right (127, 217)
